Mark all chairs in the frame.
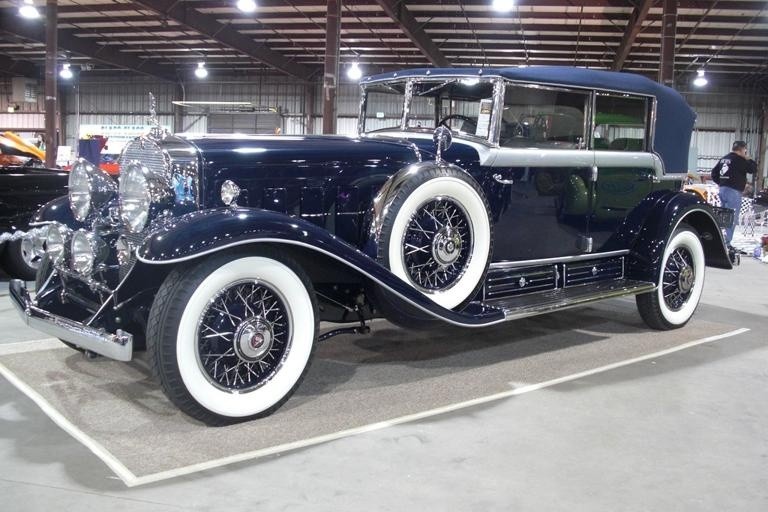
[706,187,768,237]
[462,106,587,146]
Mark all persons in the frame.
[37,133,47,152]
[711,141,757,247]
